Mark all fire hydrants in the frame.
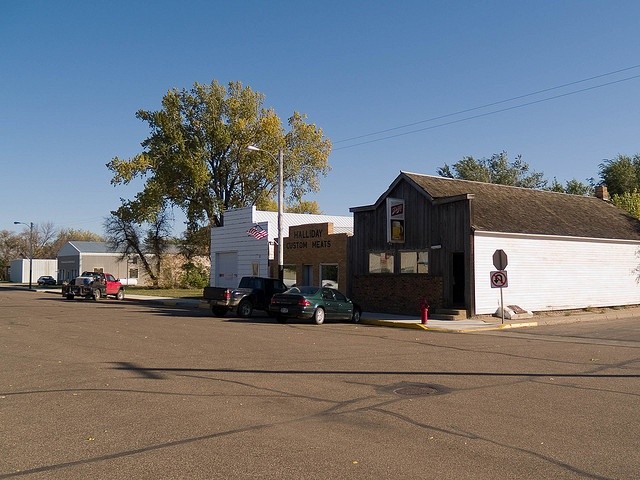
[419,298,430,323]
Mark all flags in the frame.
[247,225,268,240]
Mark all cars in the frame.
[270,286,362,325]
[38,276,56,285]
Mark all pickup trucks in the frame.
[62,271,125,300]
[204,277,287,317]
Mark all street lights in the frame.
[247,145,286,291]
[14,222,32,289]
